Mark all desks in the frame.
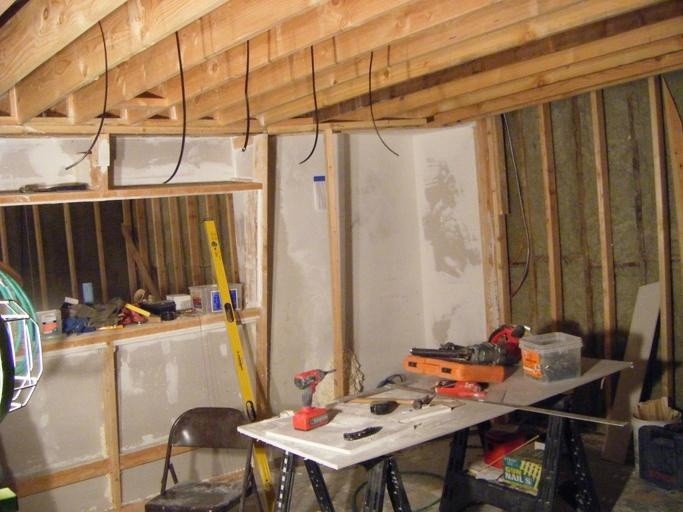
[236,340,632,509]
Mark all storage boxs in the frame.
[518,329,584,381]
[402,352,507,385]
[166,293,193,312]
[188,280,242,313]
[500,434,556,490]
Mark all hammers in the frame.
[345,397,423,410]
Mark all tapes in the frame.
[161,311,175,320]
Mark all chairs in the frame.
[141,404,255,510]
